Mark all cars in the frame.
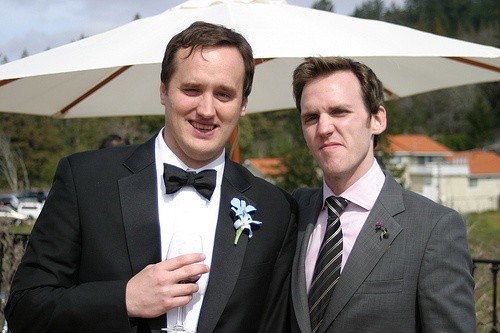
[0,190,49,227]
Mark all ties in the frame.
[308,196,350,333]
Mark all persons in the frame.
[283,56,477,333]
[4,20,299,333]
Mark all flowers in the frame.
[230,197,262,245]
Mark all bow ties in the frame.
[163,163,217,201]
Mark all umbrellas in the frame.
[0,0,500,164]
